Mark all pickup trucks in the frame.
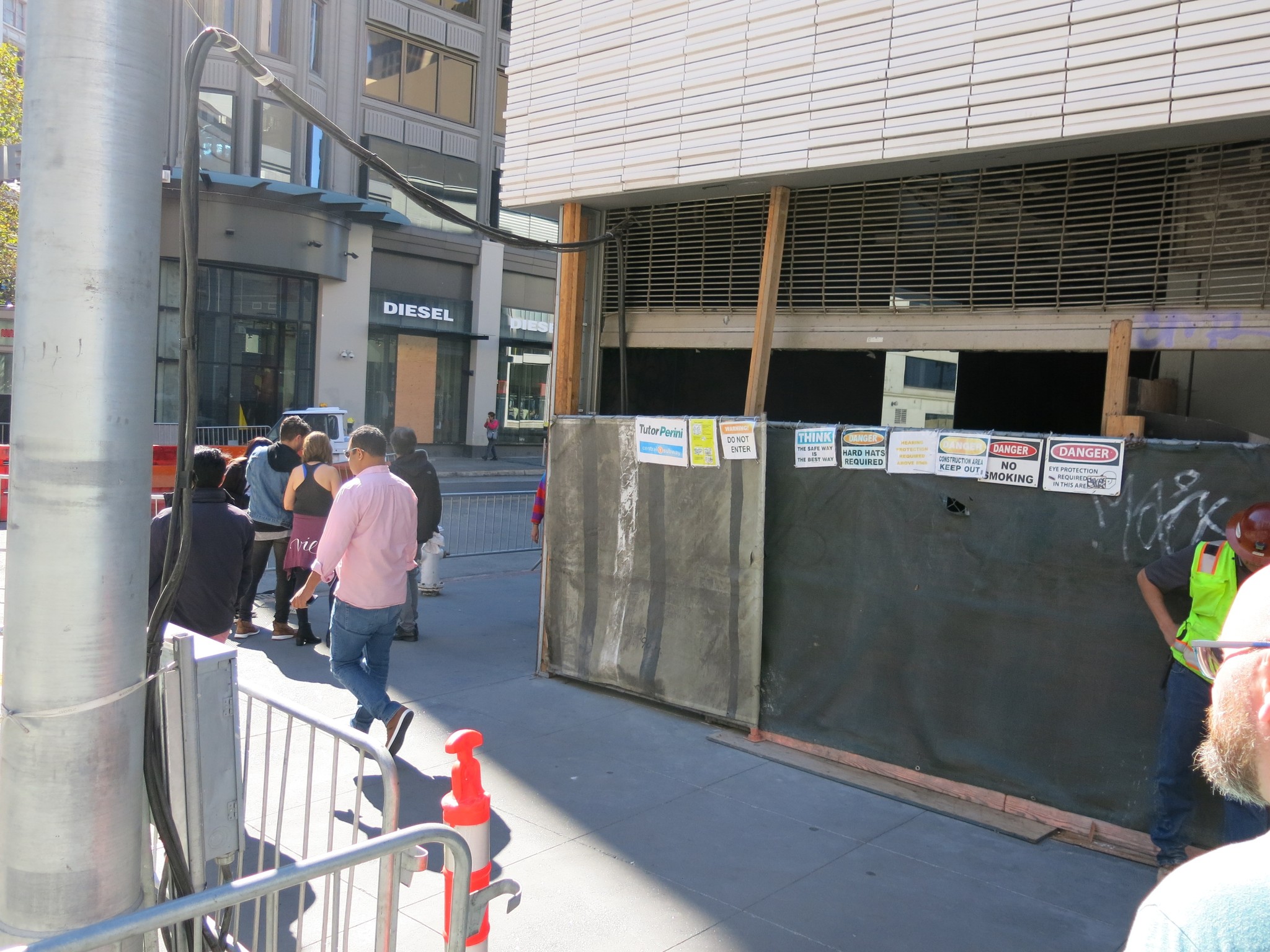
[262,404,353,467]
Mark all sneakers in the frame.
[482,456,487,460]
[272,620,299,640]
[1157,863,1183,883]
[288,605,297,615]
[385,705,414,756]
[490,457,498,461]
[234,618,260,637]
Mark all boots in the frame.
[296,621,322,646]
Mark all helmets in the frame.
[1225,502,1270,568]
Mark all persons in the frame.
[1133,503,1270,878]
[283,432,344,648]
[481,412,500,462]
[530,462,546,545]
[1123,562,1268,952]
[217,437,275,509]
[386,427,442,641]
[289,423,418,760]
[230,414,311,640]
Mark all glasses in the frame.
[1191,641,1270,680]
[343,448,365,458]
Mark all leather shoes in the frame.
[392,624,418,642]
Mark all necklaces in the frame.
[145,445,255,644]
